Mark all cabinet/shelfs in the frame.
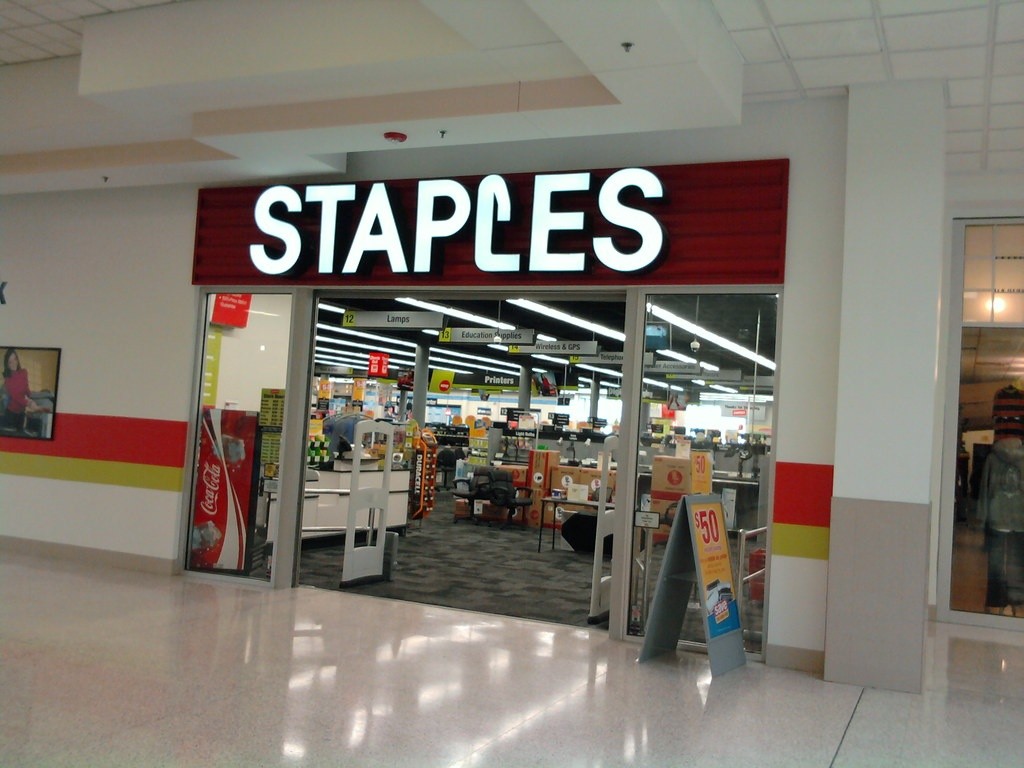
[435,434,471,457]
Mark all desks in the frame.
[538,495,615,554]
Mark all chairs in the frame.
[434,447,458,492]
[451,466,500,526]
[488,470,534,531]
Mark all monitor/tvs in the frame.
[645,321,672,351]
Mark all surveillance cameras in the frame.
[691,342,700,352]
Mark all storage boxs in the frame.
[749,548,766,601]
[455,450,691,543]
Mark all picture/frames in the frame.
[0,345,61,441]
